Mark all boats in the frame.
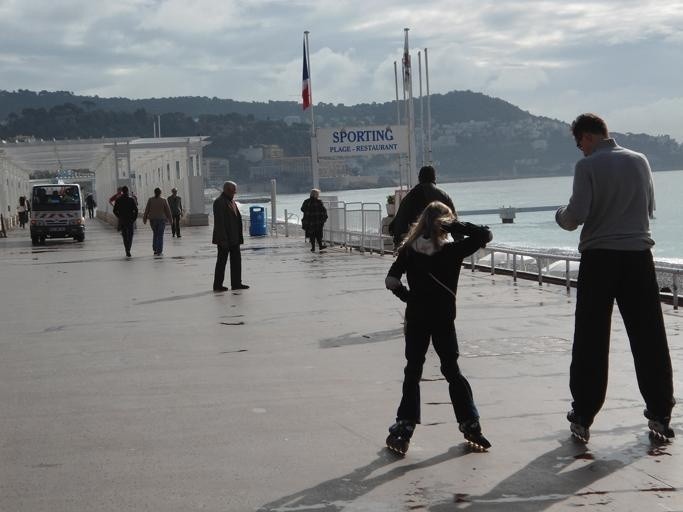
[235,197,271,203]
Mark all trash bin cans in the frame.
[249,205,267,238]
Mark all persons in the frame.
[387,166,458,253]
[210,180,250,292]
[15,188,96,228]
[300,188,328,251]
[381,199,494,443]
[107,184,183,259]
[554,112,677,428]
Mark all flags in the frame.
[300,37,311,110]
[401,29,412,94]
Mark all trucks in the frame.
[18,180,93,243]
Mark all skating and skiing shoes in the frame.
[644,410,675,438]
[567,410,594,441]
[386,433,409,457]
[464,432,490,452]
